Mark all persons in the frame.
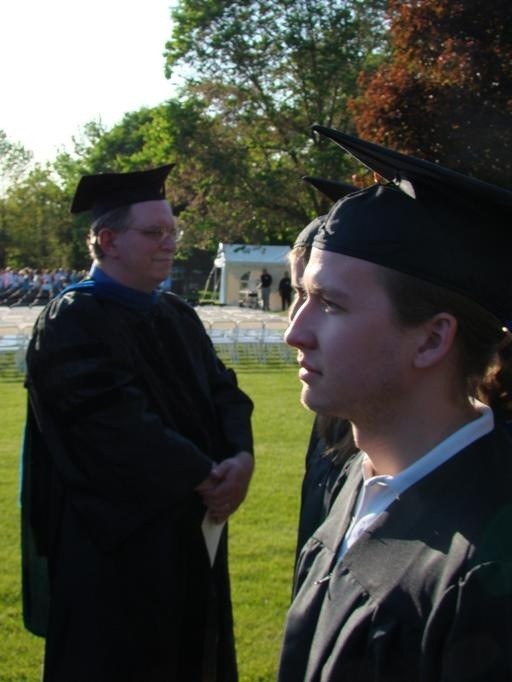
[0,267,199,307]
[275,125,510,679]
[260,267,272,310]
[279,271,294,310]
[288,176,366,599]
[23,163,254,680]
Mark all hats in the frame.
[71,164,174,213]
[292,176,362,248]
[312,125,511,319]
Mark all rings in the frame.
[226,504,231,511]
